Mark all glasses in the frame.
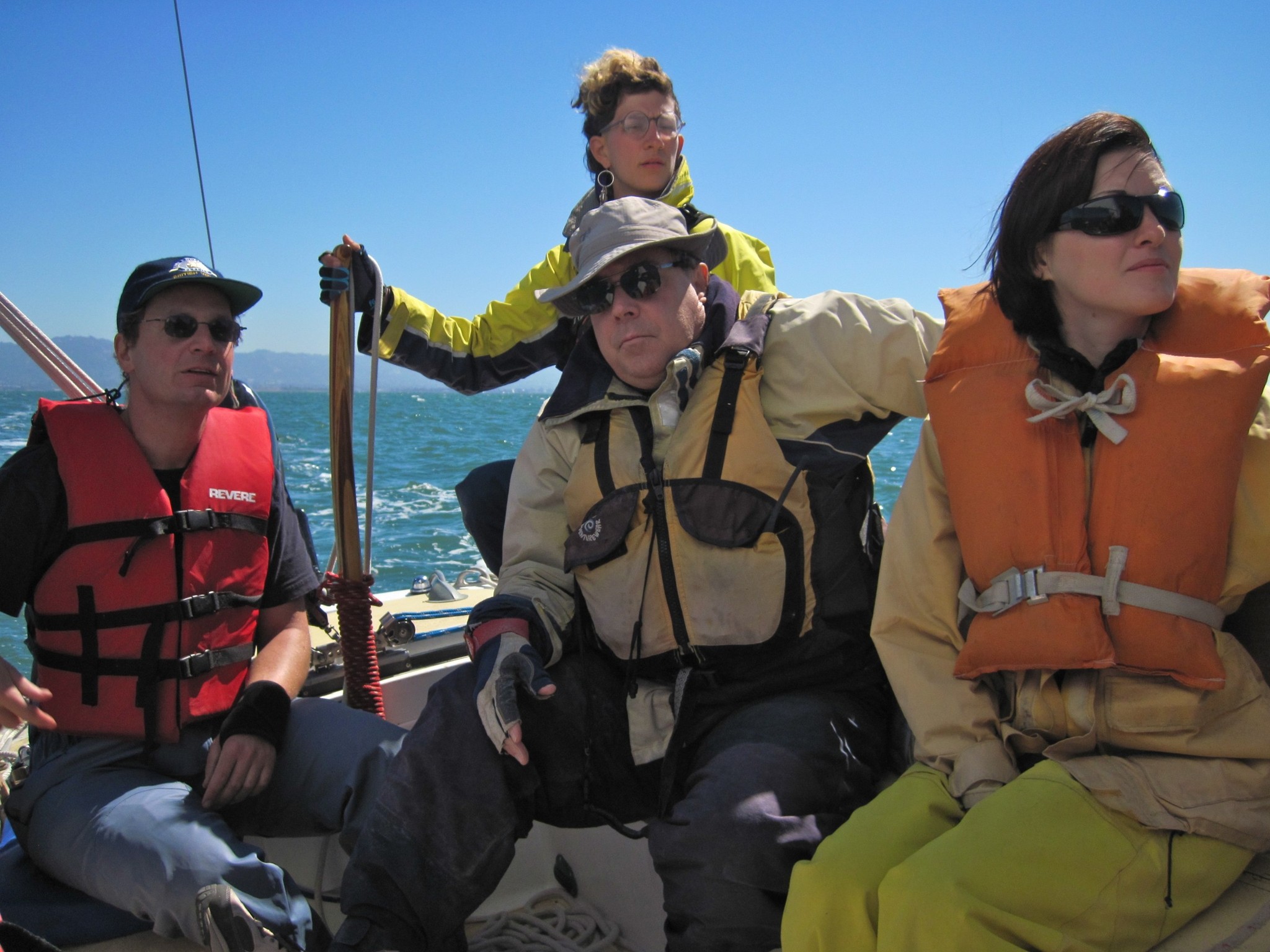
[1057,191,1184,236]
[139,313,247,344]
[598,110,687,140]
[575,260,693,315]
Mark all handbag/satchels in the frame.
[488,643,655,829]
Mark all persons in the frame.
[338,194,945,952]
[780,109,1270,951]
[318,45,775,579]
[0,256,470,951]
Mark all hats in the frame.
[115,255,261,331]
[532,195,727,304]
